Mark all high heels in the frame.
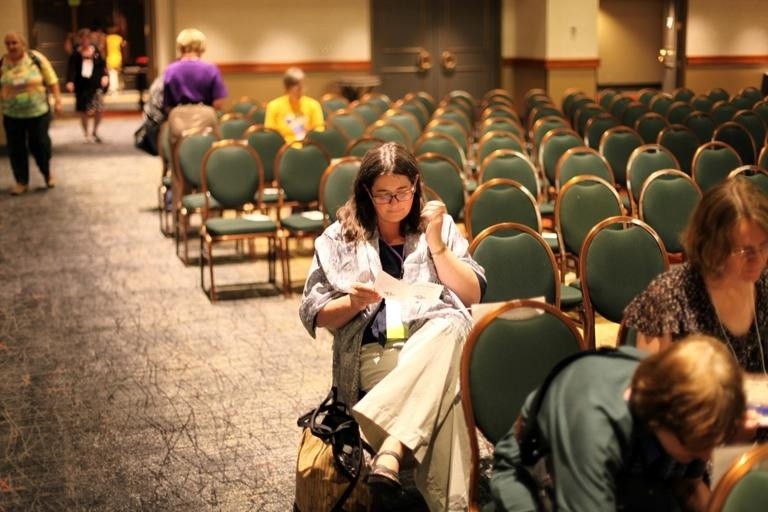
[360,449,405,495]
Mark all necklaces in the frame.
[707,285,768,380]
[379,228,412,274]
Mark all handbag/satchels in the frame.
[517,415,557,511]
[292,384,370,512]
[133,102,164,156]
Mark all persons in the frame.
[142,73,173,211]
[64,10,128,142]
[484,335,746,512]
[0,33,63,195]
[266,66,324,150]
[621,176,768,445]
[299,142,487,511]
[162,28,228,111]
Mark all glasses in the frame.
[724,240,767,259]
[359,174,417,208]
